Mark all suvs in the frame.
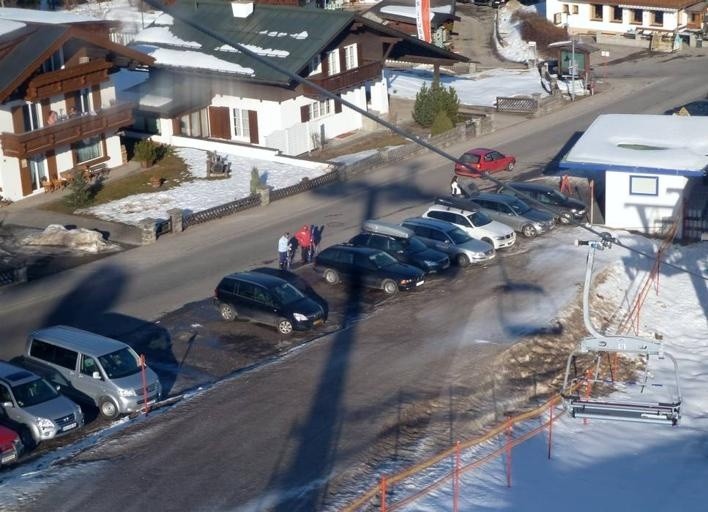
[0,360,85,451]
[213,270,325,334]
[301,182,589,293]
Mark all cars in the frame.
[0,424,23,471]
[454,148,516,178]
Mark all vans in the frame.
[23,325,162,421]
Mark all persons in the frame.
[294,224,315,264]
[277,231,292,271]
[449,176,463,199]
